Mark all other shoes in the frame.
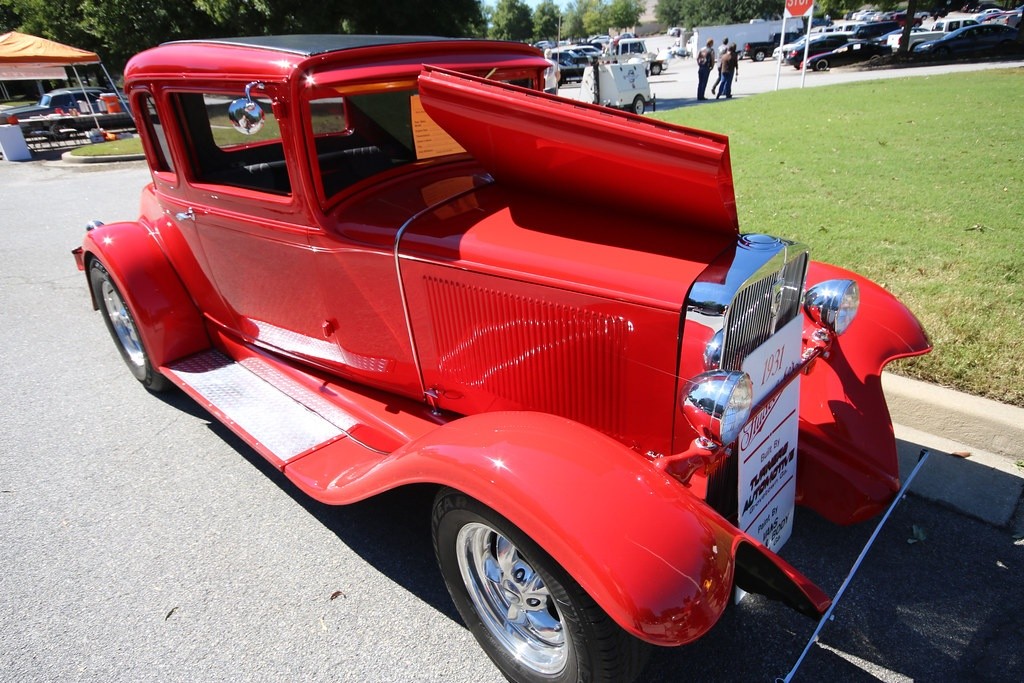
[726,96,732,98]
[712,89,715,94]
[716,97,719,99]
[703,98,708,100]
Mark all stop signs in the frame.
[784,0,814,17]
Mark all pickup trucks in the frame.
[743,31,804,62]
[886,15,985,59]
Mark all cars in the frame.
[508,27,692,86]
[0,86,160,142]
[69,34,942,683]
[912,23,1024,62]
[772,7,1024,52]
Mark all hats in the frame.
[544,49,552,59]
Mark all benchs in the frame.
[237,146,385,198]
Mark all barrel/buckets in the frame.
[99,93,122,112]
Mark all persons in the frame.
[695,37,738,101]
[542,49,562,96]
[974,28,983,37]
[239,115,253,133]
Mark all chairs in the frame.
[55,119,78,148]
[28,121,54,152]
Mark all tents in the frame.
[0,31,136,133]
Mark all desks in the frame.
[18,112,125,151]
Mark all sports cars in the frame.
[806,40,893,71]
